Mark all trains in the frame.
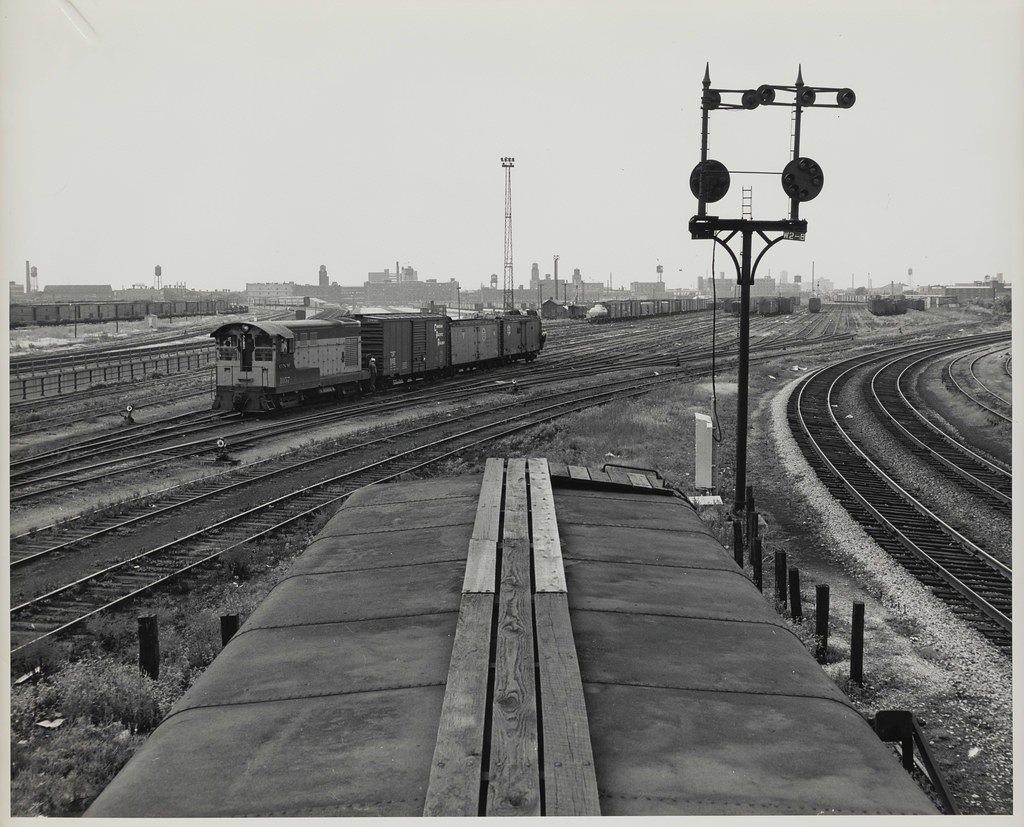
[208,298,548,422]
